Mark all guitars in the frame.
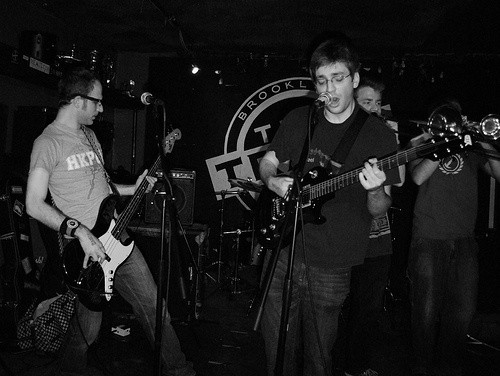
[254,123,481,250]
[59,124,182,310]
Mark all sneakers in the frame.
[344,368,380,376]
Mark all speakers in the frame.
[143,169,197,226]
[0,195,22,306]
[125,221,210,322]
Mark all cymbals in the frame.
[226,177,266,193]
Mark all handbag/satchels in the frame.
[16,289,80,352]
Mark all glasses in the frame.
[71,93,102,106]
[312,74,351,87]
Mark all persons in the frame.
[354,80,406,376]
[258,39,402,376]
[25,71,198,376]
[406,101,500,376]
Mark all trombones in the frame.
[376,101,500,157]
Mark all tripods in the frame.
[205,187,268,295]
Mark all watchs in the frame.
[66,219,78,235]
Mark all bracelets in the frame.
[60,216,81,237]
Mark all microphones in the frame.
[140,92,164,106]
[315,92,333,106]
